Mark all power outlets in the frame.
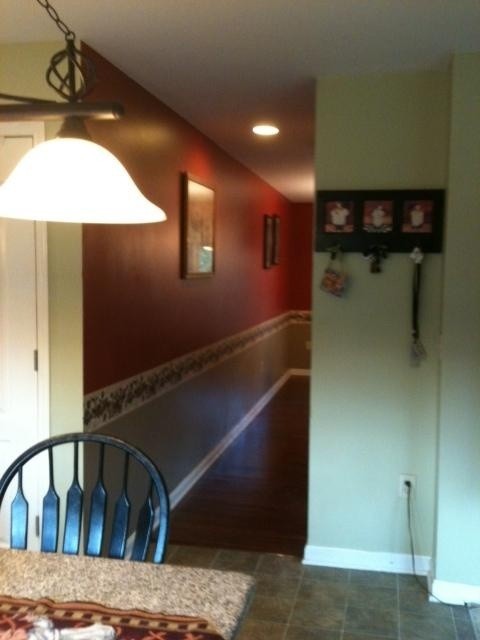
[398,474,415,495]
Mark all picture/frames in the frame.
[263,214,276,268]
[179,171,216,280]
[273,220,281,264]
[314,187,446,255]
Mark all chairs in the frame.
[0,434,167,566]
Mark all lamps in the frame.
[0,137,168,227]
